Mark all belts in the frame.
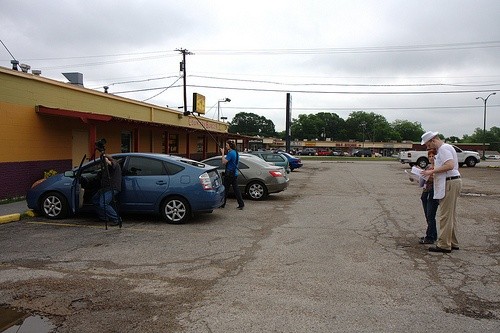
[446,176,460,180]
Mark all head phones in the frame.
[230,140,234,147]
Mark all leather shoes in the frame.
[429,246,460,253]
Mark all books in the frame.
[410,165,430,182]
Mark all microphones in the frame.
[184,111,193,116]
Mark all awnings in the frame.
[35,104,264,141]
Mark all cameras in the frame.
[96,138,107,146]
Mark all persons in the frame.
[92,155,122,227]
[217,140,246,210]
[418,132,462,252]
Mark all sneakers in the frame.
[93,216,109,221]
[109,219,122,226]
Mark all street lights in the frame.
[475,92,497,160]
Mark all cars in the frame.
[250,151,303,172]
[274,147,399,157]
[487,155,500,159]
[199,153,290,199]
[24,152,224,222]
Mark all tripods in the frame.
[96,146,123,230]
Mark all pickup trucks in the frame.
[400,145,481,167]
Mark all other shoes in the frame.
[219,204,225,208]
[237,204,244,208]
[418,237,434,244]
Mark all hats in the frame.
[420,132,438,145]
